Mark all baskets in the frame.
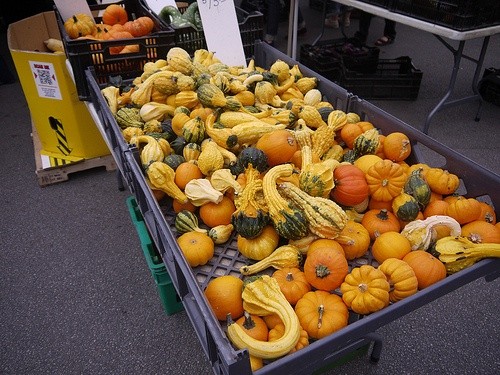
[300,39,381,80]
[144,0,265,67]
[74,56,500,372]
[325,56,423,102]
[54,0,177,102]
[389,0,500,32]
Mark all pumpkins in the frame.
[101,47,500,371]
[44,3,198,71]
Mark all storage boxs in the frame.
[6,0,500,375]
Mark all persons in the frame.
[262,0,308,49]
[354,0,395,45]
[324,0,351,29]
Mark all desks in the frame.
[329,0,500,136]
